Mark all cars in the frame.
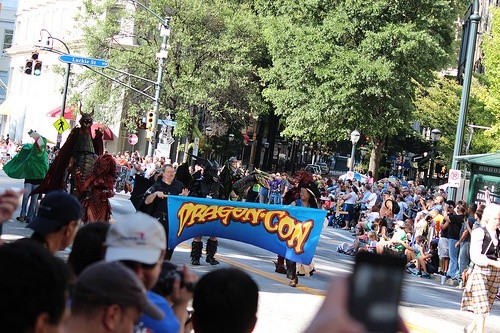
[303,161,330,176]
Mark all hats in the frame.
[396,220,406,228]
[25,191,82,231]
[104,213,168,264]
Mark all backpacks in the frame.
[385,200,399,214]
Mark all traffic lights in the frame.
[24,59,33,75]
[33,60,42,76]
[146,110,155,129]
[31,51,39,60]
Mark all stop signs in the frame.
[128,133,138,145]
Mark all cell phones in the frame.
[348,251,406,333]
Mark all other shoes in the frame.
[422,273,430,278]
[16,216,24,222]
[27,218,32,223]
[342,227,350,229]
[412,271,422,277]
[276,266,287,274]
[347,251,356,256]
[290,280,296,286]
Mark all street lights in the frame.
[426,128,442,190]
[349,128,360,172]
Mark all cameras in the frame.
[150,260,183,297]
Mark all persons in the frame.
[0,110,500,333]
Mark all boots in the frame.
[190,241,203,266]
[205,238,219,265]
